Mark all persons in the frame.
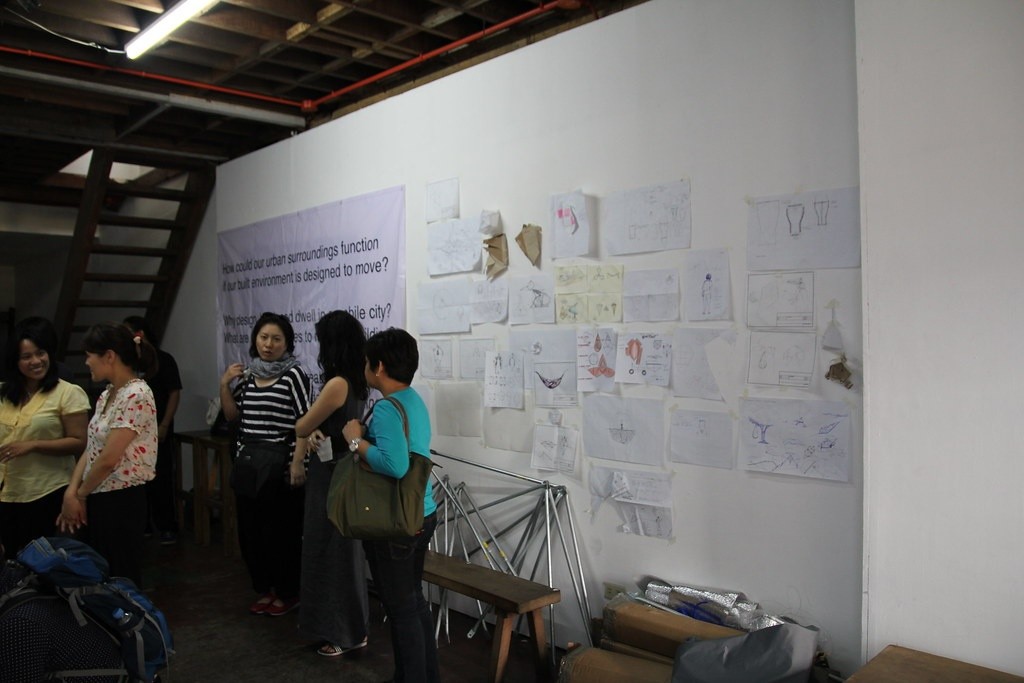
[343,326,441,681]
[56,315,181,584]
[220,313,321,616]
[295,308,372,655]
[0,316,92,569]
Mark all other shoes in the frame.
[249,591,278,613]
[265,597,302,617]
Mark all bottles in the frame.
[112,608,132,625]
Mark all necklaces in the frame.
[21,383,40,397]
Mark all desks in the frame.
[173,430,241,558]
[843,645,1024,683]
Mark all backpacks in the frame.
[16,535,175,683]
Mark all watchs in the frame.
[348,436,363,453]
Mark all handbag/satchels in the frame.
[325,398,444,540]
[228,444,293,506]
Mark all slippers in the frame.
[317,636,368,656]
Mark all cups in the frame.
[316,437,333,463]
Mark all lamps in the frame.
[124,0,219,61]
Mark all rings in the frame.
[7,452,10,455]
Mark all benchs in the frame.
[421,550,561,683]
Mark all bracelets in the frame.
[308,437,312,442]
[77,497,87,501]
